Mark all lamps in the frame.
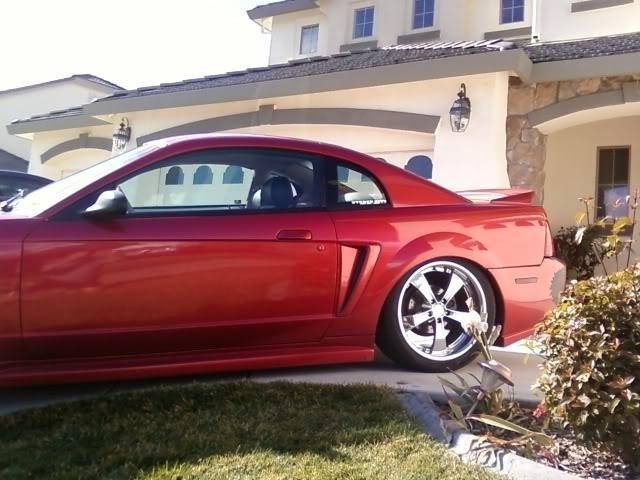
[112,116,131,154]
[449,83,471,132]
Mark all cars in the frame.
[0,170,55,203]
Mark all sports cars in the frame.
[0,133,568,385]
